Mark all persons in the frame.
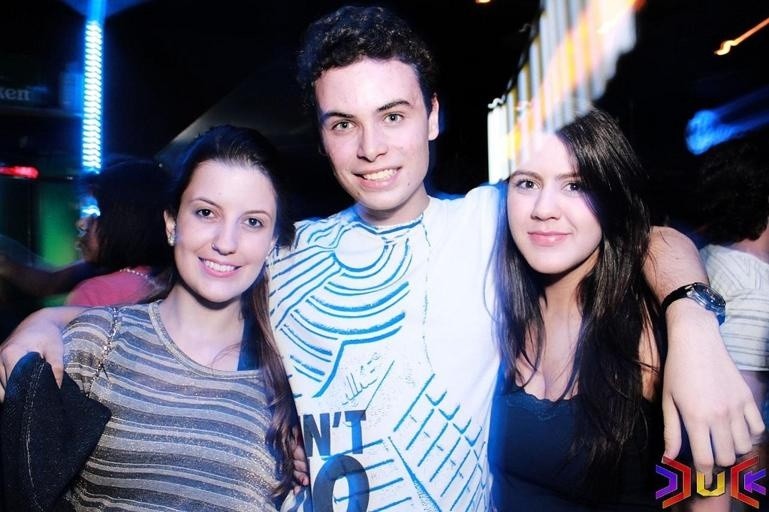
[0,7,769,512]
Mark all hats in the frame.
[3,352,112,511]
